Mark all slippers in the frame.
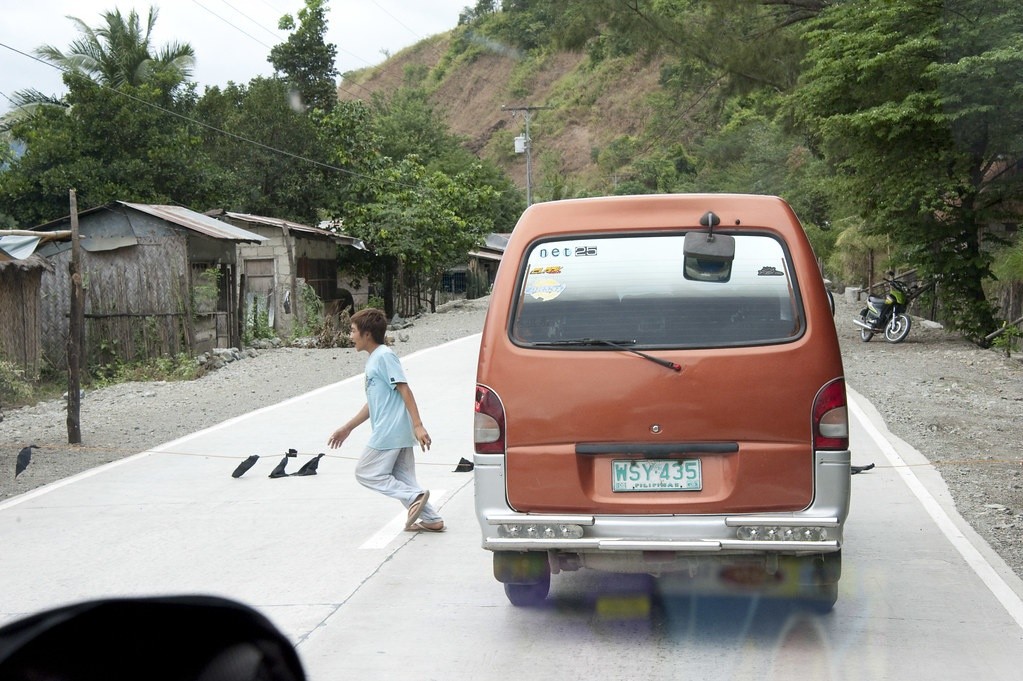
[409,523,448,532]
[408,490,430,529]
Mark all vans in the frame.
[472,192,852,616]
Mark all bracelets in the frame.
[413,423,422,429]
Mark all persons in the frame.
[327,308,450,534]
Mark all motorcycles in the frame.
[852,270,919,344]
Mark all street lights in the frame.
[513,135,533,209]
